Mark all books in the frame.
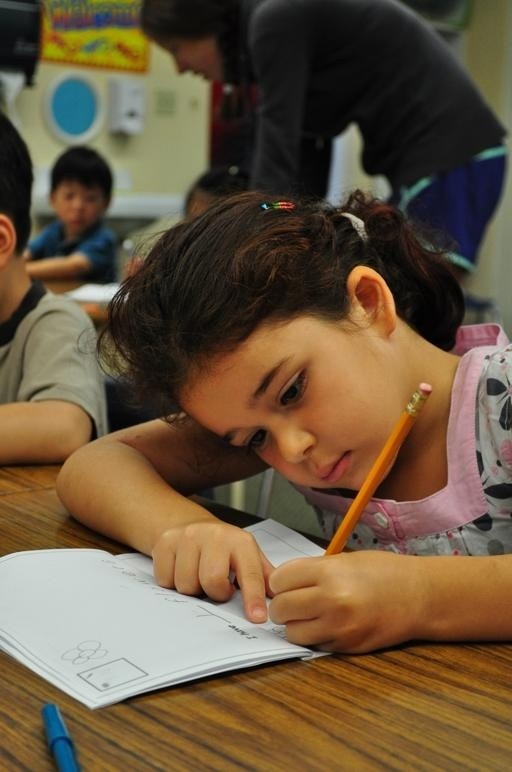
[0,517,332,713]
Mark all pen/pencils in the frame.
[41,703,81,772]
[325,382,432,555]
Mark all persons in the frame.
[54,188,512,656]
[0,112,109,466]
[22,146,120,284]
[124,167,247,277]
[138,0,510,292]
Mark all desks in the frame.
[44,282,109,327]
[1,463,512,772]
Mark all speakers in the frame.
[0,1,41,68]
